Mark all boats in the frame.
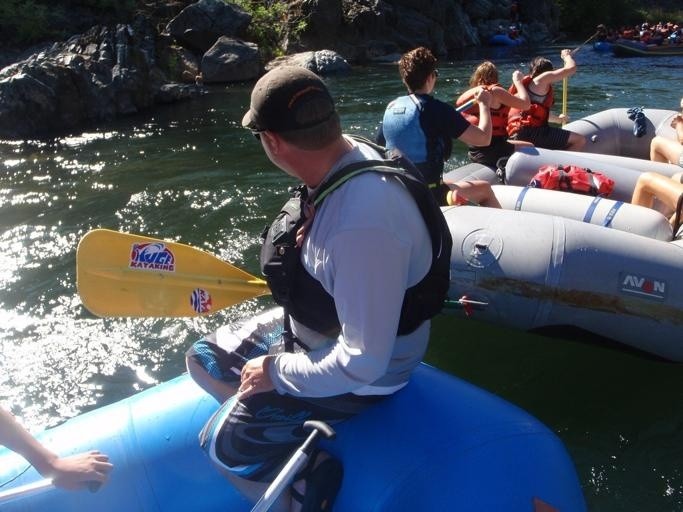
[0,356,587,512]
[487,31,528,48]
[437,104,683,364]
[592,36,682,57]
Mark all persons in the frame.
[374,47,501,213]
[648,114,682,167]
[456,55,530,166]
[185,63,456,511]
[496,20,525,41]
[589,16,683,51]
[0,402,112,495]
[628,168,682,231]
[499,46,587,155]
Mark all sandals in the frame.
[290,448,343,512]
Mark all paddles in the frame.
[75,229,490,318]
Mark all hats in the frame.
[242,65,335,132]
